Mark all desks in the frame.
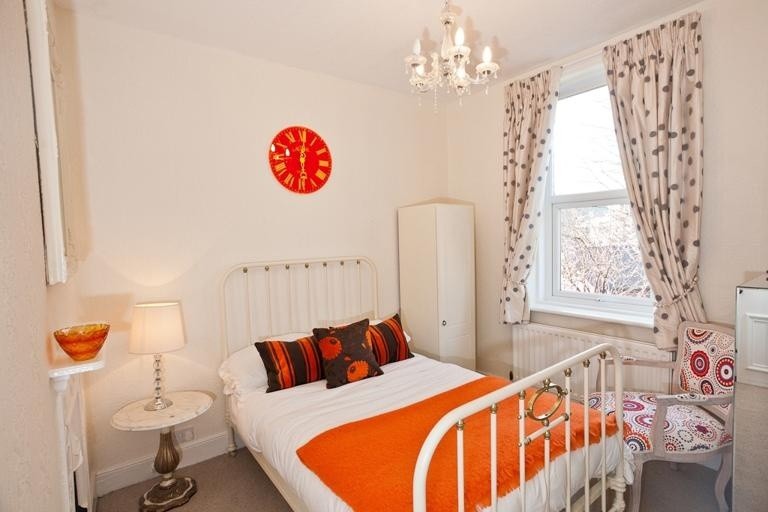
[108,391,214,512]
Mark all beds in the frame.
[219,256,626,512]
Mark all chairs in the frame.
[579,321,736,512]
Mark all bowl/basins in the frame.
[53,323,110,363]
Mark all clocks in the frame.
[269,126,332,194]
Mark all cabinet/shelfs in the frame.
[397,203,476,372]
[731,271,768,512]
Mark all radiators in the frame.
[512,322,676,401]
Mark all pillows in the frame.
[255,336,326,393]
[369,318,411,343]
[312,318,384,389]
[368,313,415,367]
[218,333,310,403]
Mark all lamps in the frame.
[128,303,185,411]
[400,0,500,115]
